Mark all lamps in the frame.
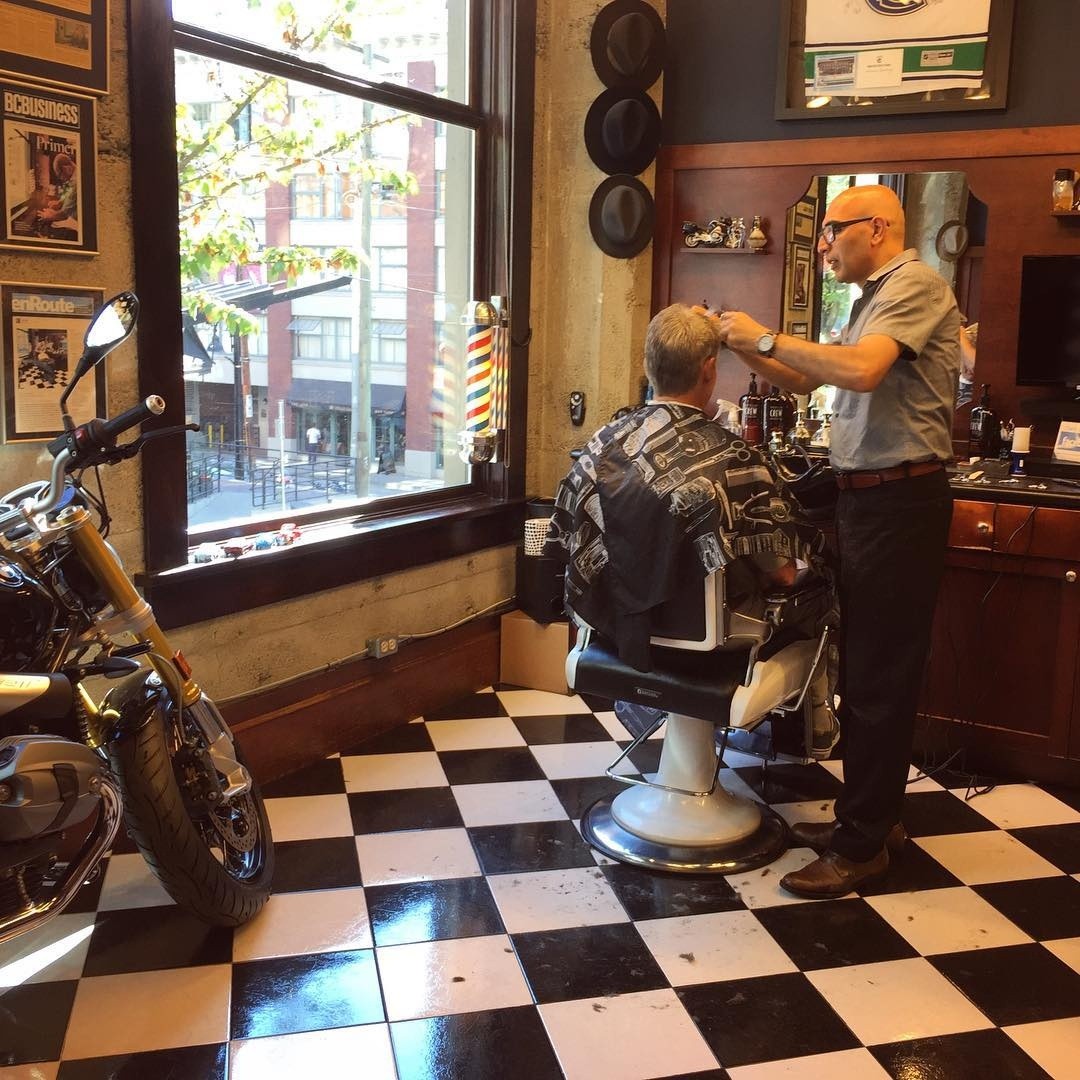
[428,293,467,458]
[464,295,508,467]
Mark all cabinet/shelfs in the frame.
[909,499,1080,786]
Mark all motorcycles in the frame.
[0,294,273,991]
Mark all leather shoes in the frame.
[789,822,905,858]
[778,840,889,898]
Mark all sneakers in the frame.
[808,709,841,761]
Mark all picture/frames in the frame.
[0,76,101,256]
[775,0,1014,121]
[0,280,109,445]
[0,0,111,97]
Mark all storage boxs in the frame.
[500,609,579,697]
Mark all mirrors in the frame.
[778,170,989,421]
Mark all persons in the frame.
[685,182,970,902]
[305,420,323,466]
[621,299,842,761]
[36,152,80,234]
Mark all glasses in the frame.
[817,217,891,245]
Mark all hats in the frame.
[583,87,663,177]
[589,0,667,98]
[588,173,656,259]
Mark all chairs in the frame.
[563,474,837,876]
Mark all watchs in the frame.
[754,328,783,362]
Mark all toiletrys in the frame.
[737,371,794,458]
[967,384,1000,463]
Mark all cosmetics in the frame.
[736,370,763,444]
[761,384,789,443]
[965,381,999,457]
